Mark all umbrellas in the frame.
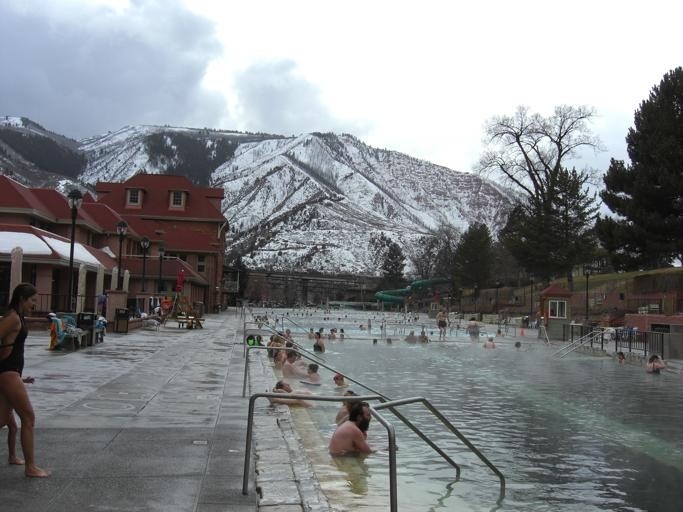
[175,268,184,293]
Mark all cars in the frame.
[585,326,641,341]
[638,303,660,314]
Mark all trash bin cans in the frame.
[194,301,203,317]
[77,312,95,346]
[235,302,240,307]
[113,308,130,333]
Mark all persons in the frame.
[617,351,625,364]
[264,348,398,459]
[250,300,541,348]
[0,374,35,465]
[0,282,50,476]
[646,354,665,374]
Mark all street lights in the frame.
[474,282,476,315]
[458,288,463,314]
[117,221,128,291]
[66,190,84,312]
[158,246,165,291]
[528,273,535,317]
[494,279,499,307]
[140,235,150,292]
[582,264,591,321]
